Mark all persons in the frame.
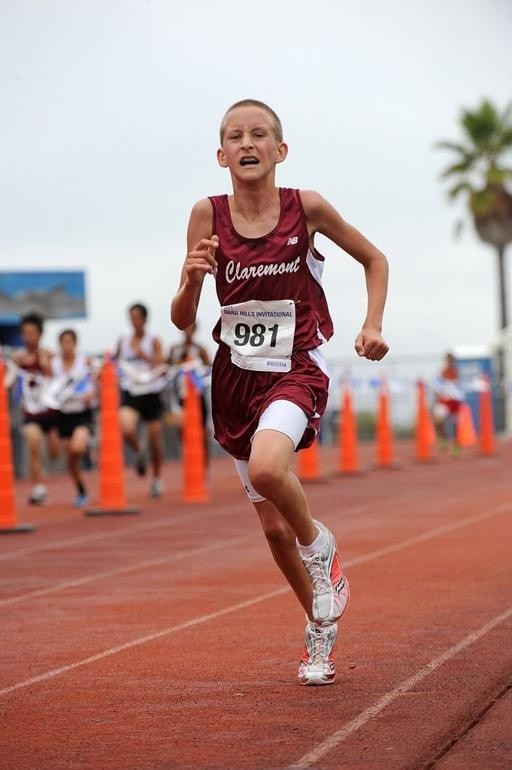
[167,321,211,483]
[2,313,63,508]
[110,304,166,498]
[433,352,465,458]
[40,327,97,508]
[171,98,389,684]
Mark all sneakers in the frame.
[22,445,166,510]
[293,517,352,623]
[293,616,343,688]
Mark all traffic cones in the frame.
[335,386,366,479]
[0,354,35,533]
[452,399,477,449]
[298,433,332,484]
[478,381,499,457]
[409,377,442,466]
[79,353,141,516]
[178,372,212,503]
[372,391,400,471]
